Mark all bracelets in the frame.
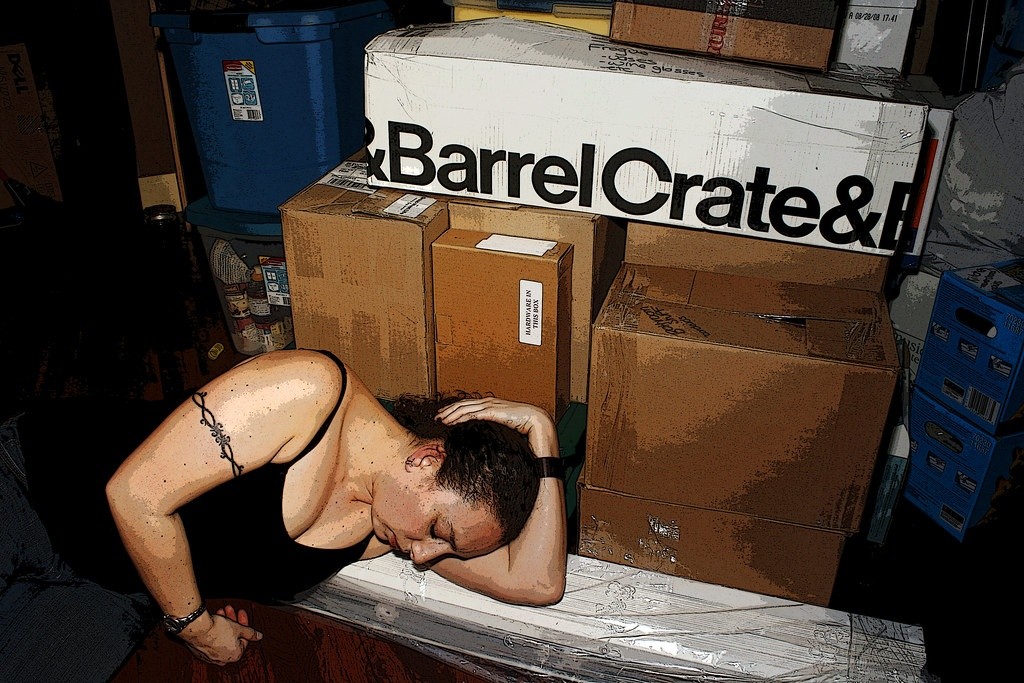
[535,457,562,482]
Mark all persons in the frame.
[0,347,568,683]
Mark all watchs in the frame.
[162,601,206,634]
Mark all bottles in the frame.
[248,273,276,322]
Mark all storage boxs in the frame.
[610,1,840,72]
[625,222,889,293]
[0,42,72,211]
[185,198,296,354]
[150,1,399,214]
[365,18,928,256]
[903,386,1024,541]
[835,0,917,74]
[868,368,911,545]
[444,0,612,39]
[448,198,626,405]
[279,145,448,403]
[585,263,900,534]
[576,468,846,607]
[914,261,1024,436]
[431,229,574,427]
